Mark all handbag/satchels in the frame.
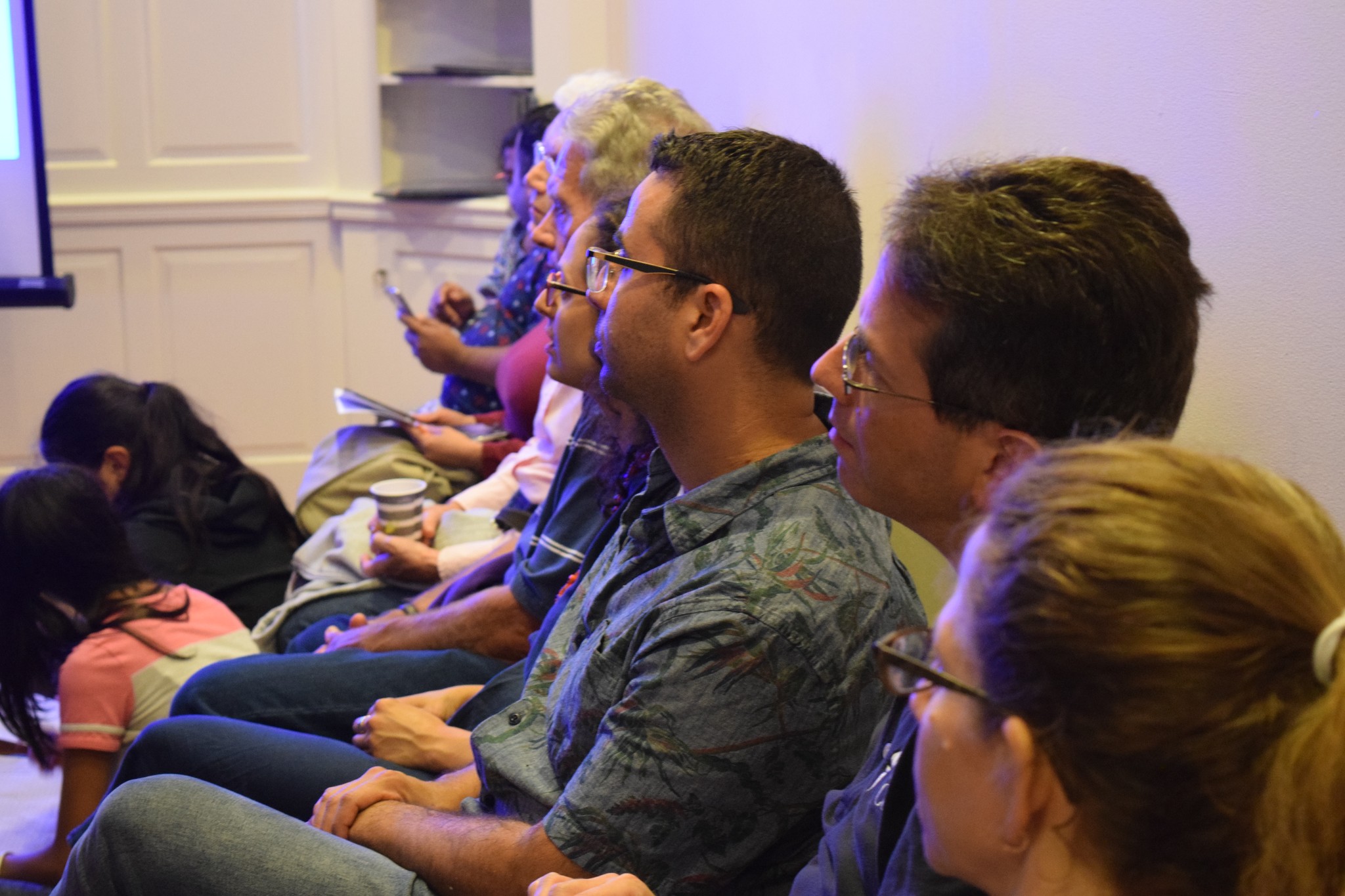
[294,423,478,538]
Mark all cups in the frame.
[369,477,427,543]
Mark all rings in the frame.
[358,716,365,733]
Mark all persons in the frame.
[0,462,264,896]
[48,129,932,896]
[39,373,311,634]
[65,71,717,847]
[522,153,1215,896]
[907,434,1345,896]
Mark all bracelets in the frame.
[396,601,418,615]
[0,848,15,869]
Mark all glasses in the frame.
[842,333,983,415]
[546,272,586,305]
[533,142,555,175]
[872,627,1004,710]
[585,247,750,316]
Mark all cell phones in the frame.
[387,287,414,317]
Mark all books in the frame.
[333,386,435,428]
